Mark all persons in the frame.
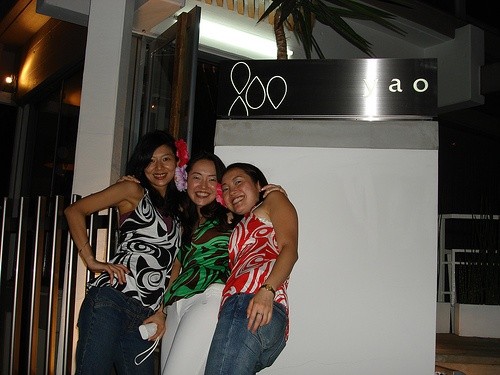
[117,152,287,375]
[65,129,184,375]
[204,163,298,375]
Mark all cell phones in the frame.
[139,323,158,339]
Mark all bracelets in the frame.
[79,242,88,253]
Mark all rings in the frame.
[257,312,264,316]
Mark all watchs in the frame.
[261,284,275,295]
